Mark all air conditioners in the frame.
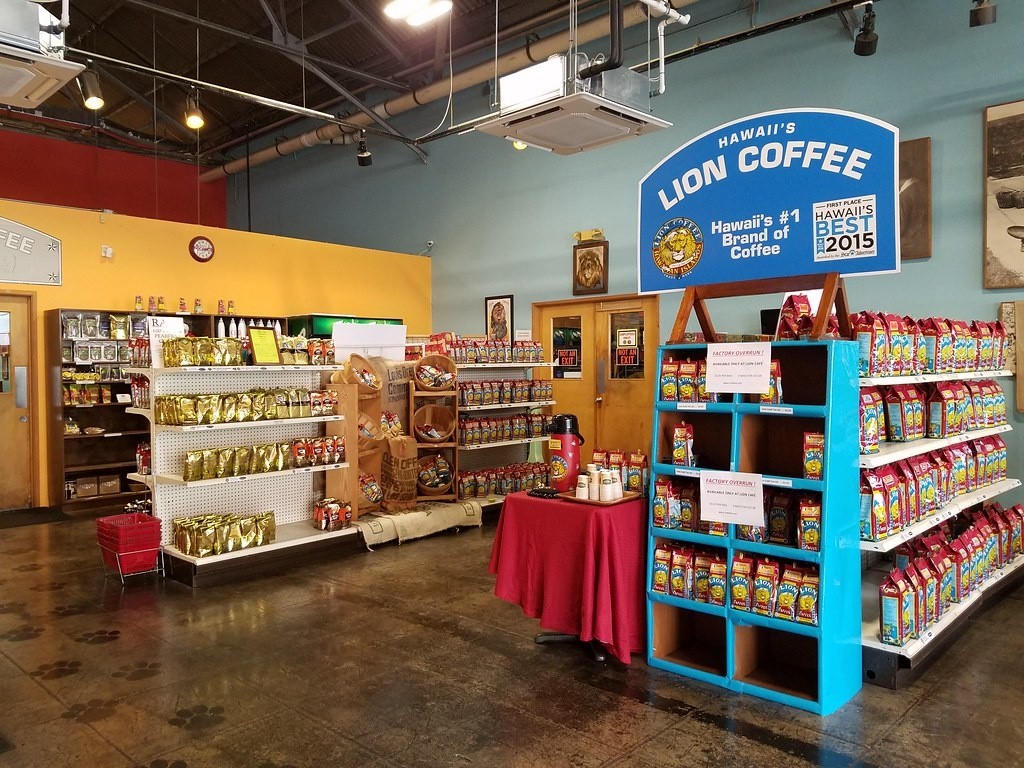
[0,0,86,108]
[475,55,673,156]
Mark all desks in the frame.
[504,487,650,660]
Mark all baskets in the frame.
[96,512,164,574]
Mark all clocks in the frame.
[189,235,215,262]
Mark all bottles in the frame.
[229,318,237,337]
[218,318,225,338]
[238,318,281,338]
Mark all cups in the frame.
[576,464,624,503]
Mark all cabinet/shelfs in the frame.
[43,308,288,511]
[648,340,1024,715]
[122,342,557,586]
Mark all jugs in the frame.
[546,414,585,492]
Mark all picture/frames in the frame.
[573,240,609,294]
[983,98,1024,289]
[485,294,514,347]
[899,136,931,259]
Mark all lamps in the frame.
[75,60,105,109]
[357,129,372,166]
[853,1,879,56]
[970,0,996,27]
[184,84,205,128]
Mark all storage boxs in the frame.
[96,514,162,573]
